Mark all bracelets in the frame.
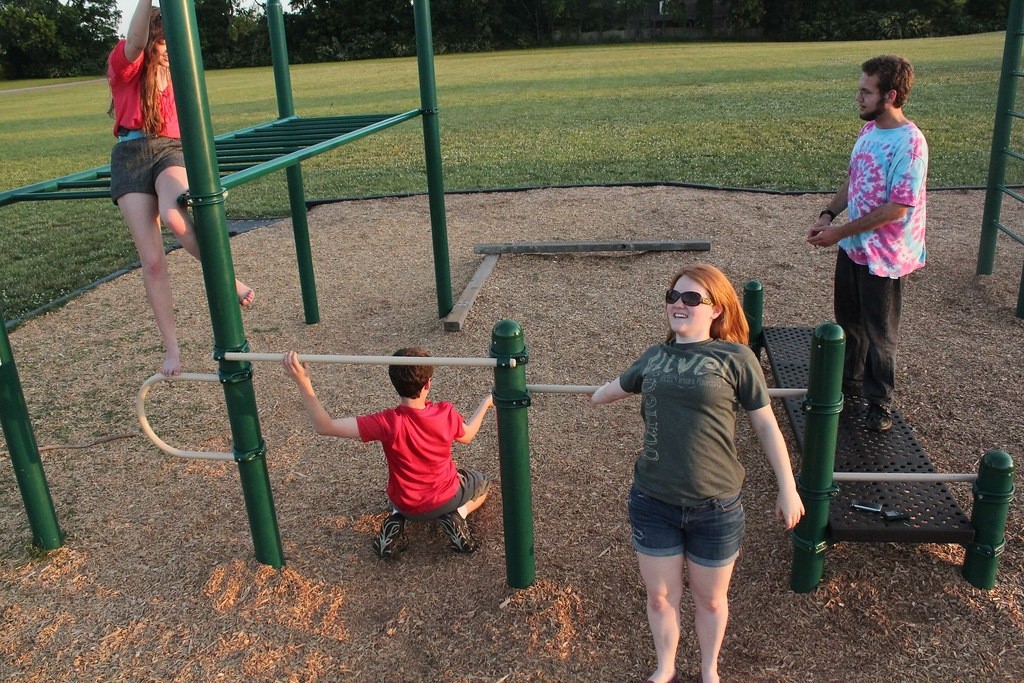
[818,210,835,222]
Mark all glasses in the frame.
[666,290,714,306]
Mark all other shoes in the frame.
[698,671,722,683]
[644,668,677,683]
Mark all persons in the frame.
[586,262,808,683]
[101,0,258,379]
[798,49,931,434]
[279,344,498,559]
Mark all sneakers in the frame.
[437,511,476,553]
[373,513,405,558]
[866,400,892,430]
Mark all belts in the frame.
[119,129,145,142]
